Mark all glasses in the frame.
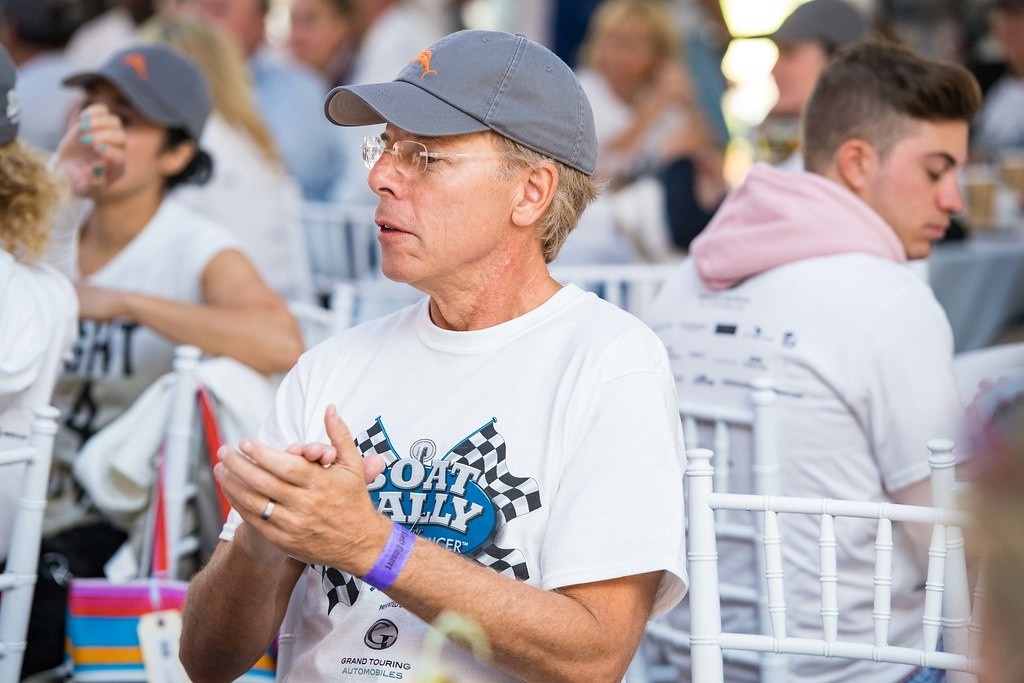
[361,136,502,176]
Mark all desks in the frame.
[927,236,1024,352]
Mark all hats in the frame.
[766,0,863,51]
[0,45,21,146]
[63,45,208,141]
[323,29,598,177]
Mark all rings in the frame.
[263,500,275,518]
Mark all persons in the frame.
[0,0,1024,683]
[178,29,689,683]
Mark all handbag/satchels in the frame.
[66,387,278,683]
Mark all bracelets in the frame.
[364,521,415,589]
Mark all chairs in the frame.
[0,180,983,683]
